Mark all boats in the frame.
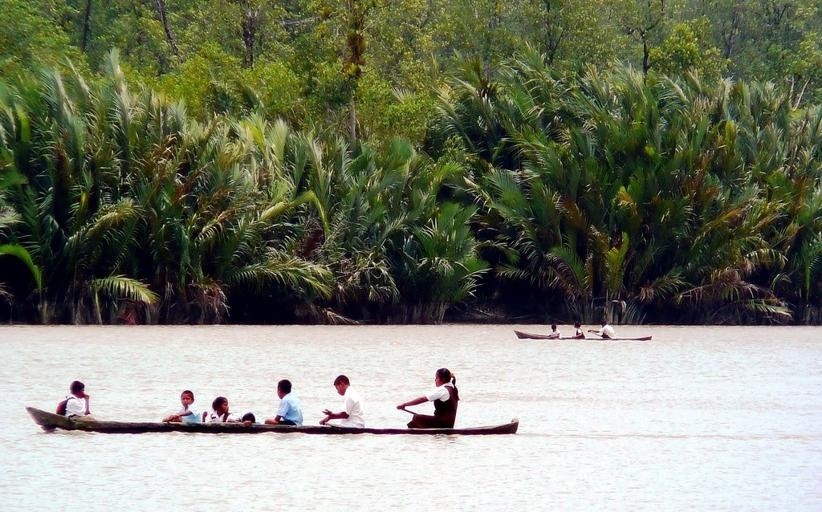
[27,407,519,434]
[515,331,652,340]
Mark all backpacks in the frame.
[56,396,75,416]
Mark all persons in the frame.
[57,381,96,421]
[397,367,460,428]
[573,321,585,339]
[162,390,202,422]
[318,375,365,429]
[202,396,237,422]
[264,380,303,425]
[548,324,561,339]
[242,412,261,425]
[590,318,616,338]
